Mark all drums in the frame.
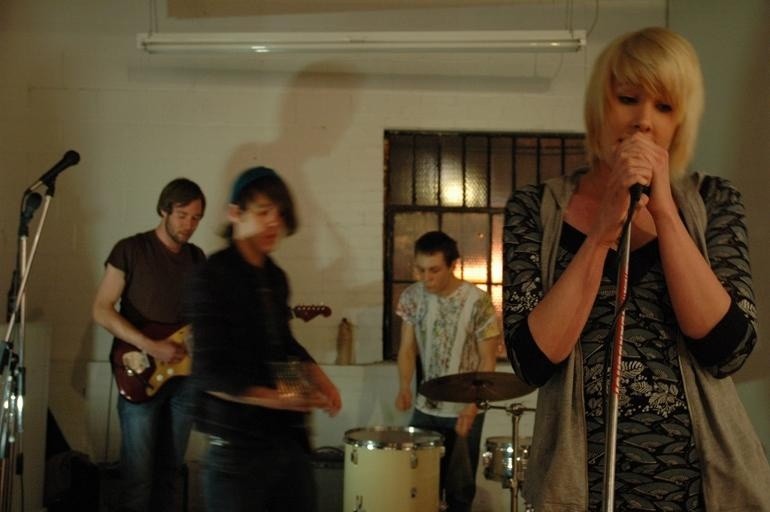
[340,424,447,511]
[481,434,536,490]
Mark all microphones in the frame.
[17,192,42,237]
[23,149,81,194]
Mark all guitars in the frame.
[106,300,334,407]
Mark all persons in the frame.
[395,228,501,510]
[502,24,760,509]
[91,177,206,511]
[197,166,342,510]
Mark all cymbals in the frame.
[416,369,538,405]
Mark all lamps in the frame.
[139,30,589,56]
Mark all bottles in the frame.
[336,317,352,366]
